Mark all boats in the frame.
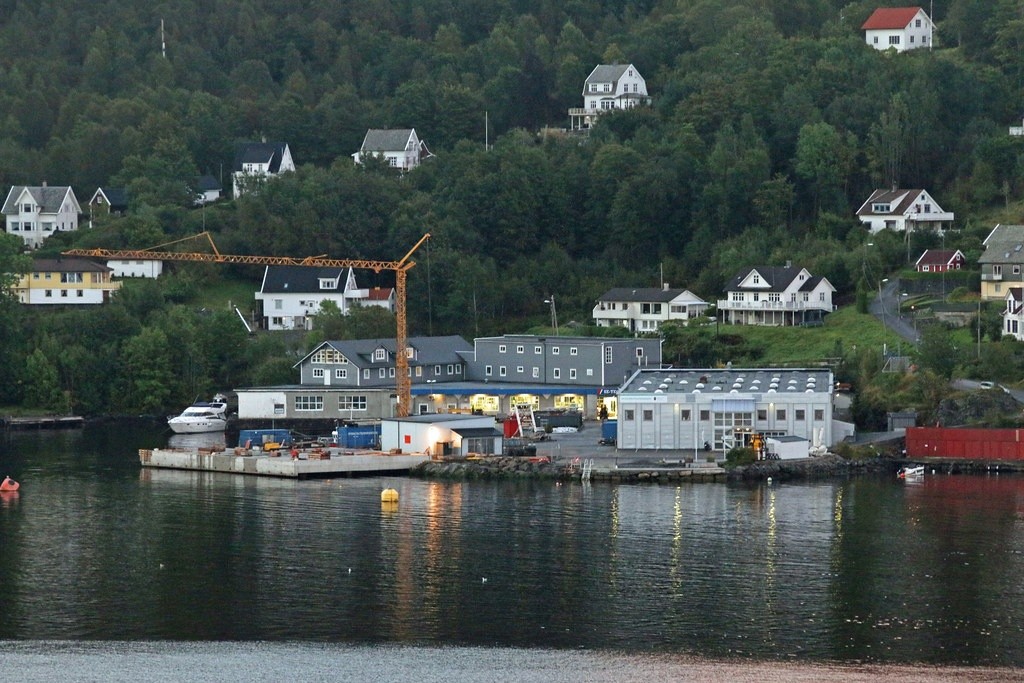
[167,394,227,433]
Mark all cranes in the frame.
[61,233,429,418]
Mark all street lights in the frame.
[879,279,888,325]
[544,300,555,334]
[897,294,907,326]
[862,242,873,263]
